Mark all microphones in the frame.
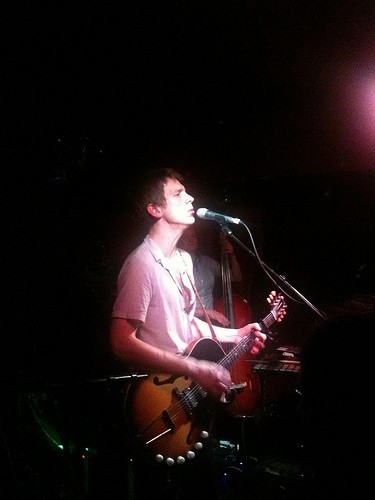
[196,208,242,225]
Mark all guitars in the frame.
[121,289,290,465]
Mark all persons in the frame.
[108,166,266,398]
[176,225,243,328]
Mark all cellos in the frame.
[197,195,265,421]
